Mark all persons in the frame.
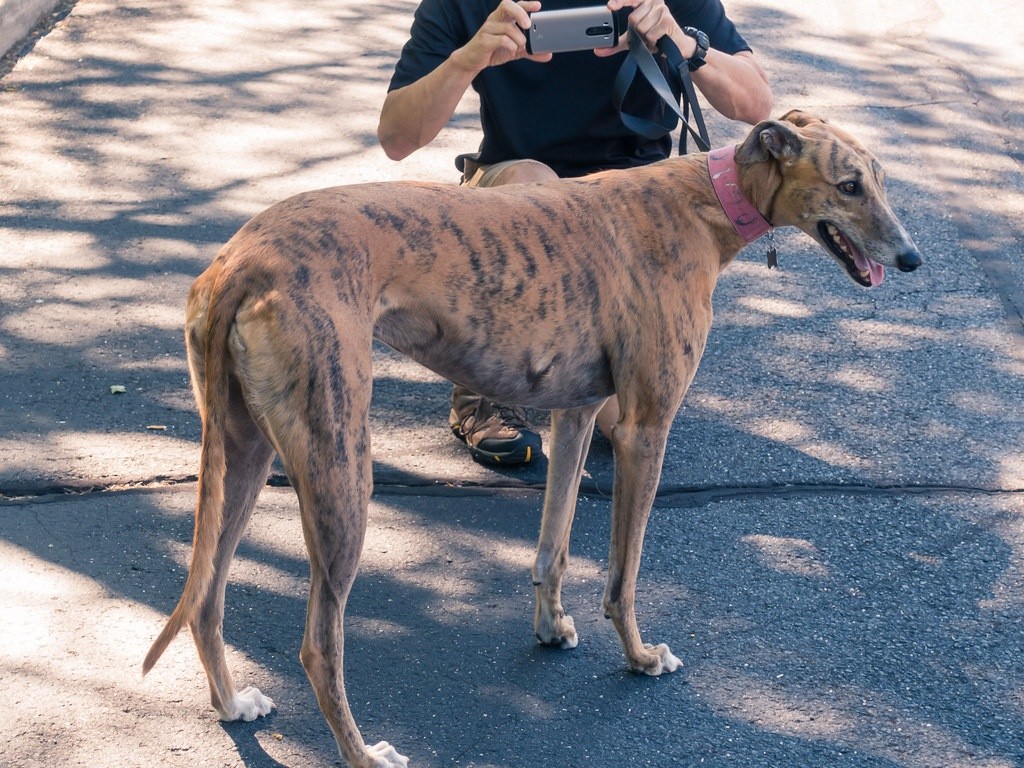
[378,0,771,467]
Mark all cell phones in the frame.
[526,5,620,55]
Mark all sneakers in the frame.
[448,384,542,465]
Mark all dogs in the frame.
[144,108,923,768]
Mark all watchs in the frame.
[681,26,709,72]
